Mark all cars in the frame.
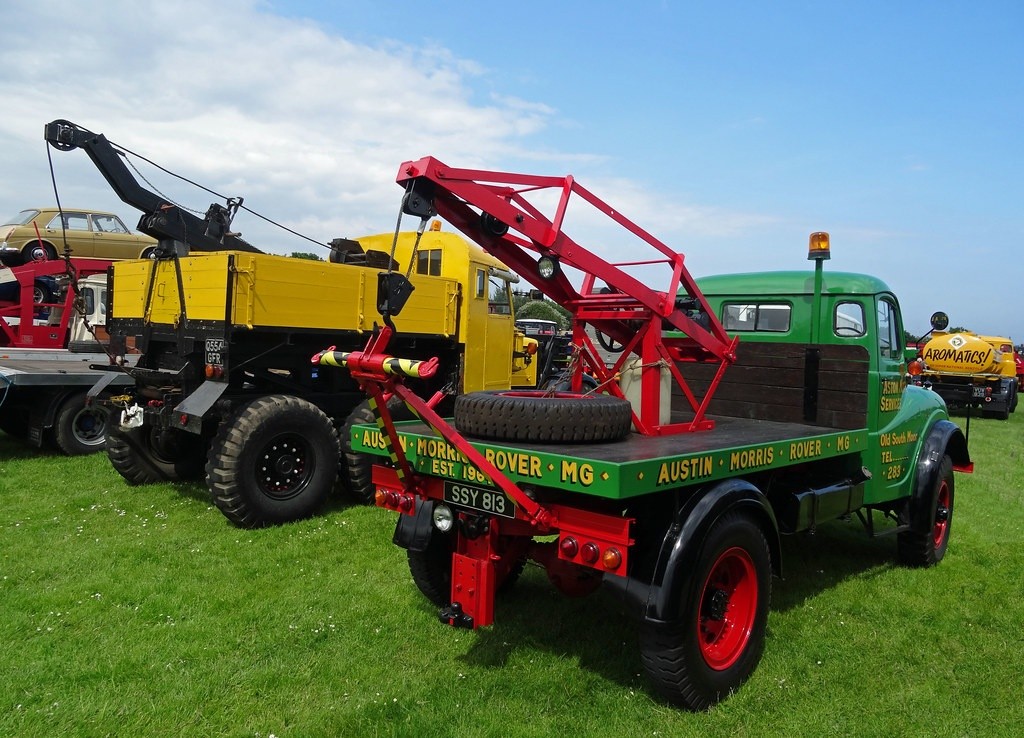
[0,205,164,266]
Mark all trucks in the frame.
[914,332,1019,420]
[308,155,975,711]
[40,119,542,532]
